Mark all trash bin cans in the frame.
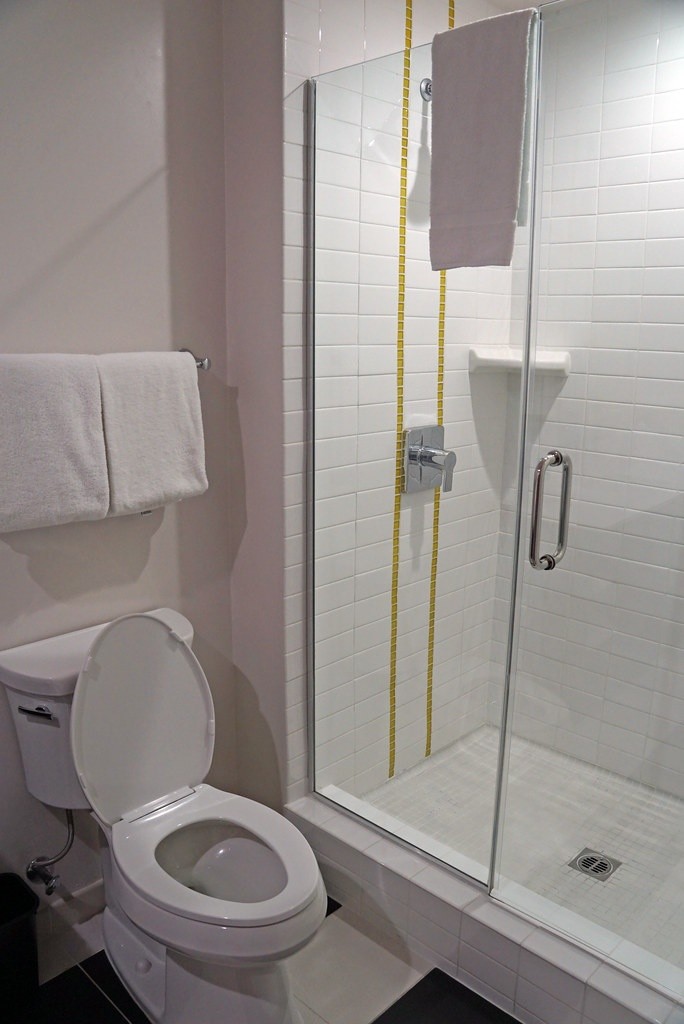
[0,872,40,1024]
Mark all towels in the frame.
[95,351,210,517]
[1,353,109,534]
[427,7,538,273]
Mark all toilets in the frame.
[0,607,327,1024]
